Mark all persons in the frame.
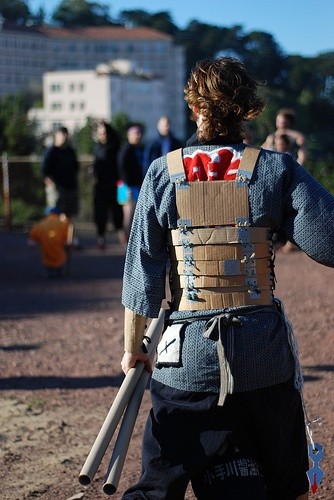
[92,121,127,252]
[120,55,334,500]
[117,123,147,245]
[142,116,185,171]
[40,127,80,249]
[258,109,306,255]
